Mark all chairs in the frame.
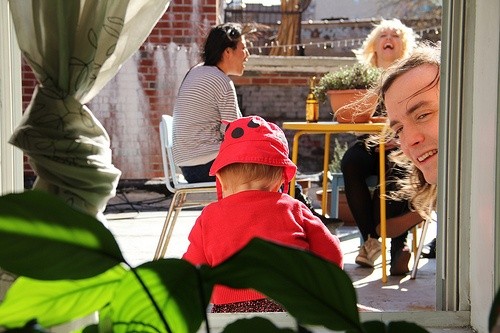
[152,114,319,263]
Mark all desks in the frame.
[282,120,416,283]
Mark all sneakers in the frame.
[355,233,382,267]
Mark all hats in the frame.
[209,116,297,182]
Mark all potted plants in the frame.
[316,138,377,227]
[313,62,386,124]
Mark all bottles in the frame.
[305,77,320,123]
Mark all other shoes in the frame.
[389,246,412,276]
[417,238,437,259]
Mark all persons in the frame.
[173,24,344,232]
[340,19,415,273]
[381,45,441,214]
[183,115,342,313]
